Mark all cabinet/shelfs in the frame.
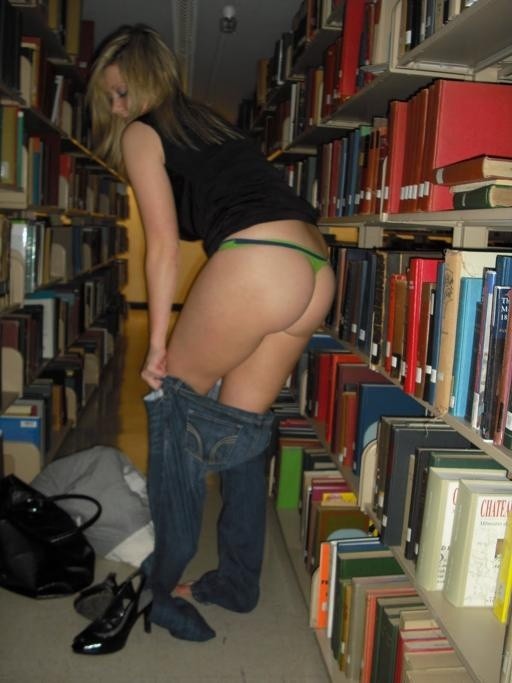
[0,0,128,484]
[270,0,510,680]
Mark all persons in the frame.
[78,21,338,616]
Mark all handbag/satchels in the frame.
[1,474,102,598]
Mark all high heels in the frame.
[72,571,153,655]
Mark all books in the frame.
[232,0,510,682]
[1,0,129,490]
